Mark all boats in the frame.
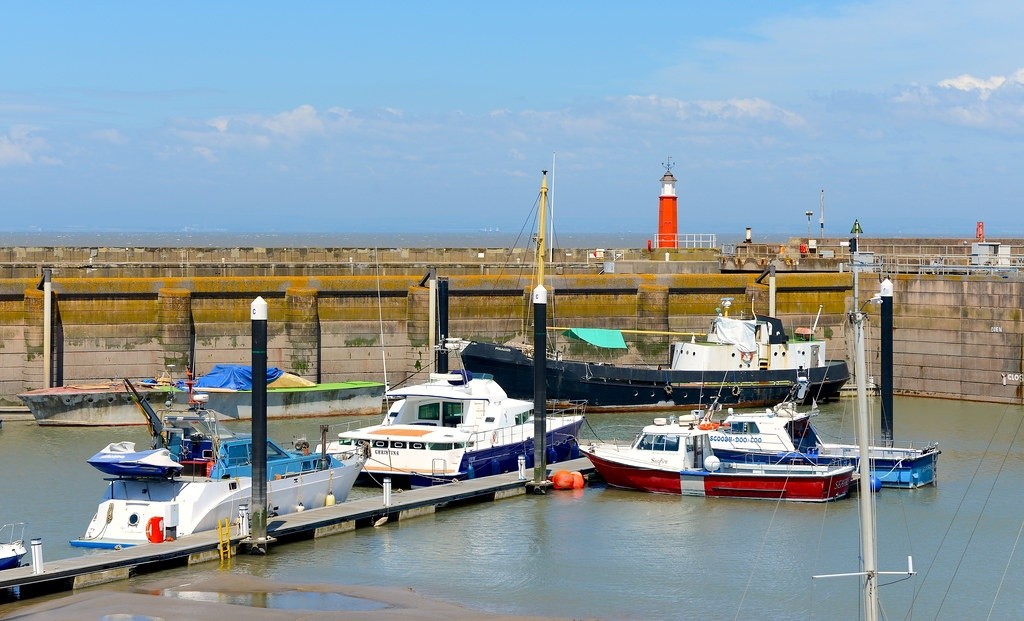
[312,367,588,488]
[63,375,370,555]
[717,401,942,490]
[577,410,855,504]
[430,304,852,403]
[0,522,29,572]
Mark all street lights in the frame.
[806,211,814,238]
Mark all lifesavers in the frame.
[594,251,606,260]
[699,421,730,429]
[741,351,753,361]
